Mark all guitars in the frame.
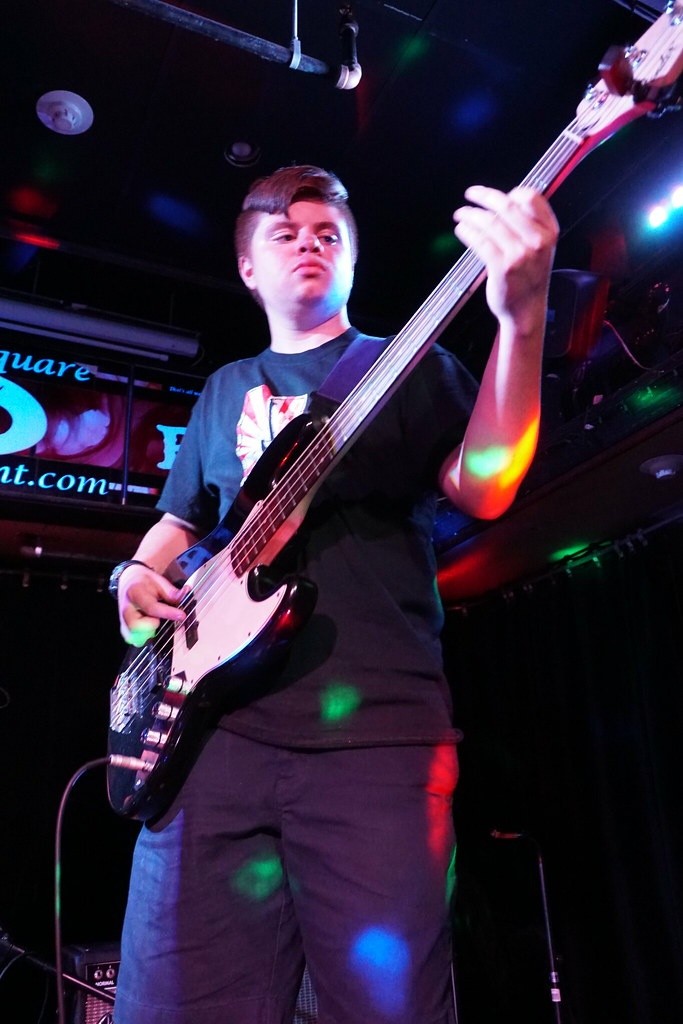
[100,1,681,833]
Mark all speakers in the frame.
[48,942,321,1024]
[542,269,612,370]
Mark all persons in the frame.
[108,163,558,1024]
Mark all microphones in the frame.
[486,828,525,841]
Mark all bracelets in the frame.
[110,561,156,598]
